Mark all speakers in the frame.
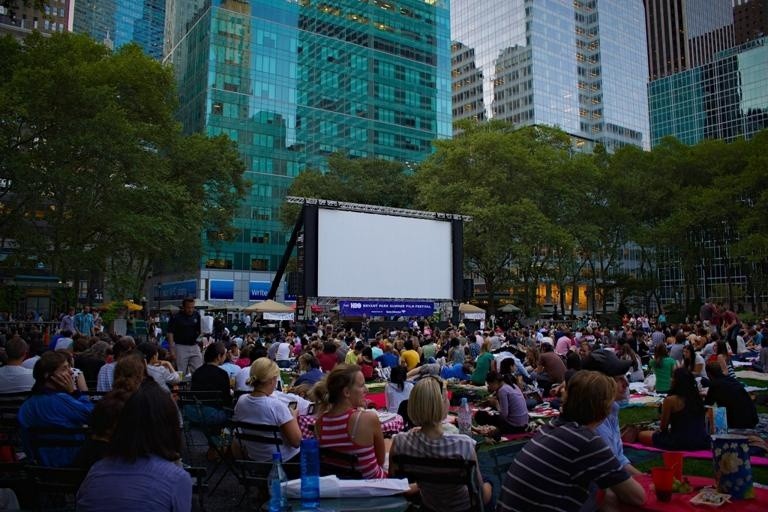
[464,279,473,298]
[289,272,304,295]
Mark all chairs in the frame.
[0,324,768,509]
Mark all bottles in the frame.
[456,397,474,437]
[229,372,236,386]
[265,452,289,512]
[298,439,322,509]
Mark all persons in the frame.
[1,300,768,512]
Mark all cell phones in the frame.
[288,402,297,411]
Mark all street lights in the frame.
[583,289,591,321]
[158,281,162,318]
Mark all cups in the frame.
[662,452,683,481]
[652,466,675,502]
[284,384,289,392]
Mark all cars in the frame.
[543,303,571,321]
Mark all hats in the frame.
[31,351,68,391]
[585,349,632,376]
